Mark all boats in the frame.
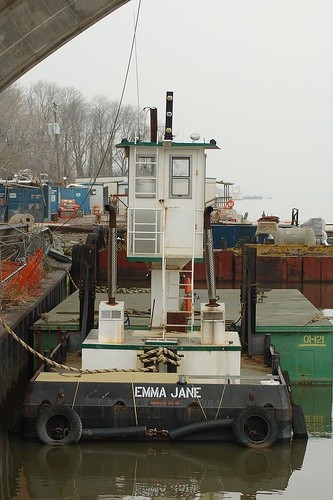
[17,439,308,499]
[20,89,308,449]
